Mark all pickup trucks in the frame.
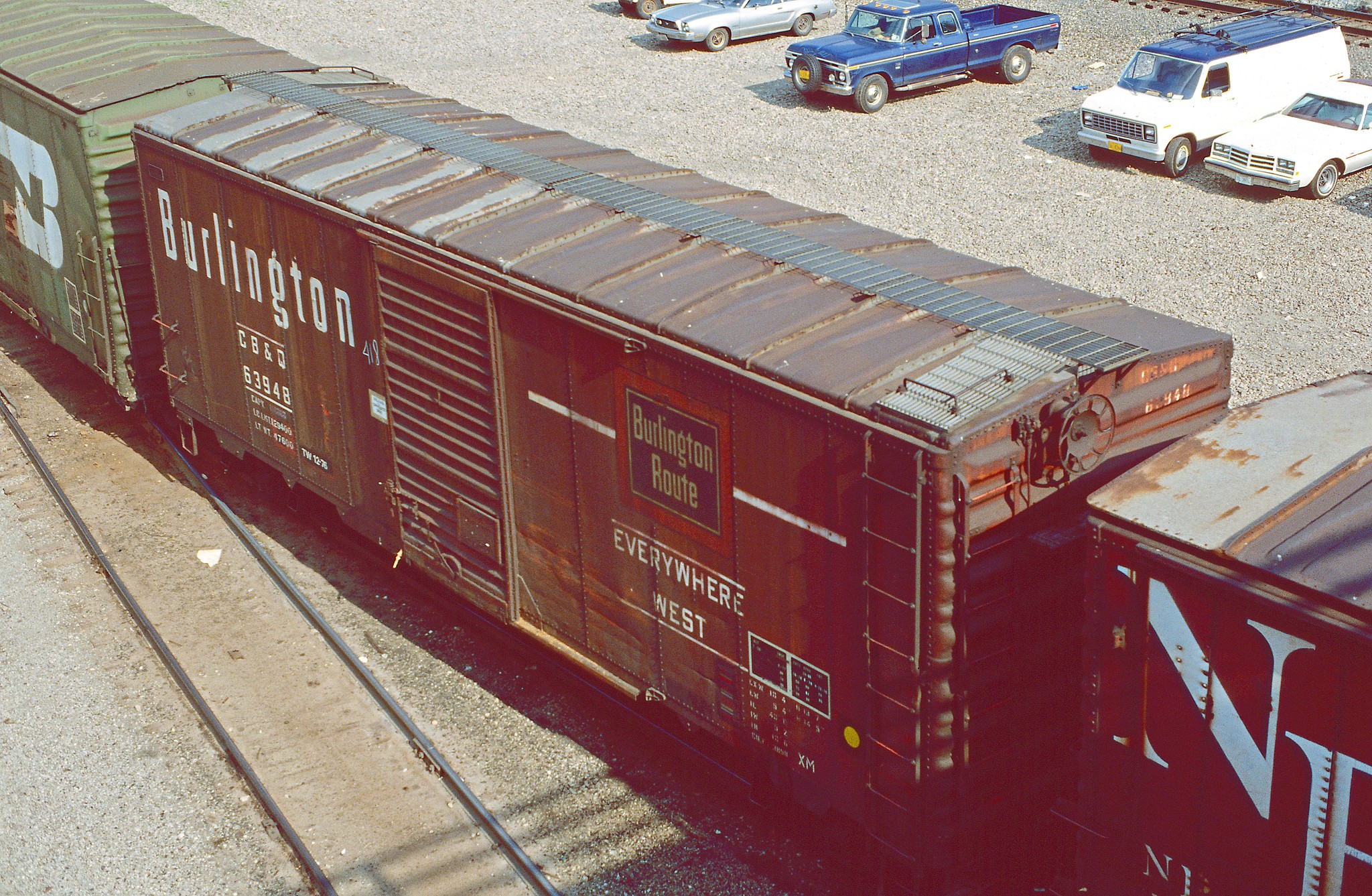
[783,0,1061,115]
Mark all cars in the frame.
[1204,78,1372,200]
[617,0,837,52]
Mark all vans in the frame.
[1076,0,1352,180]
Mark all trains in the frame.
[0,0,1371,896]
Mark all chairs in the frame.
[876,16,887,31]
